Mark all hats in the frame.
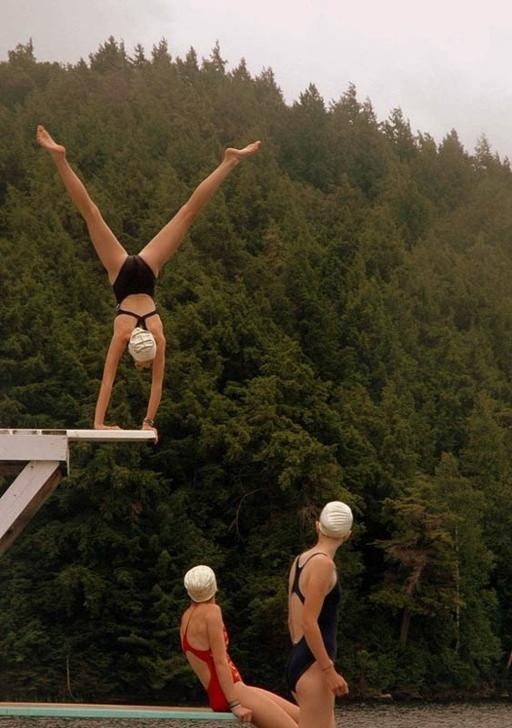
[319,501,353,536]
[128,327,157,362]
[183,564,218,603]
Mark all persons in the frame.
[288,501,353,728]
[37,124,261,445]
[180,564,300,728]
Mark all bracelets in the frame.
[144,417,155,426]
[228,698,241,709]
[322,661,335,671]
